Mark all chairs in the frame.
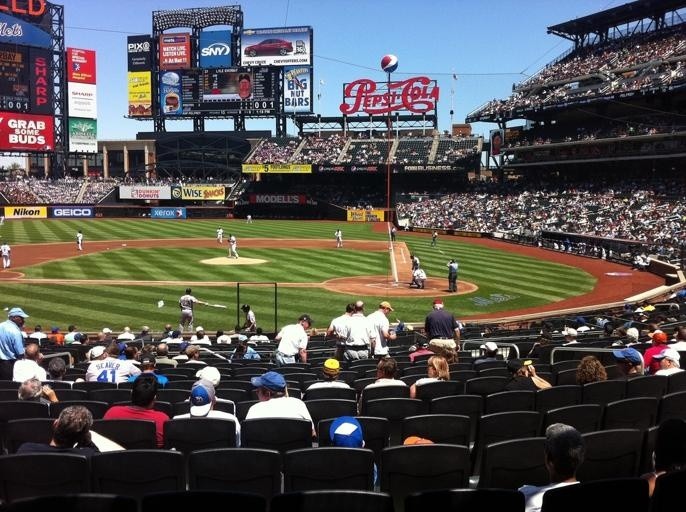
[0,296,686,511]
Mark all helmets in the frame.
[241,304,250,310]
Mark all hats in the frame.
[561,317,681,363]
[189,358,434,447]
[91,346,107,358]
[380,301,394,312]
[103,328,112,334]
[299,314,314,323]
[433,299,443,304]
[480,342,498,353]
[507,358,533,371]
[9,308,29,318]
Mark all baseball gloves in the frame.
[228,239,231,243]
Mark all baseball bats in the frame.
[208,303,227,309]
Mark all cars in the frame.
[245,39,292,57]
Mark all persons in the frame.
[246,130,479,232]
[1,175,252,223]
[391,227,397,241]
[431,231,439,245]
[1,287,465,493]
[227,233,239,259]
[216,225,224,244]
[1,242,11,268]
[518,423,586,511]
[234,73,253,99]
[473,285,685,393]
[479,25,685,272]
[335,229,343,248]
[640,418,684,510]
[76,230,83,250]
[446,259,458,292]
[409,256,427,289]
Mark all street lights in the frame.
[449,74,458,137]
[317,79,324,138]
